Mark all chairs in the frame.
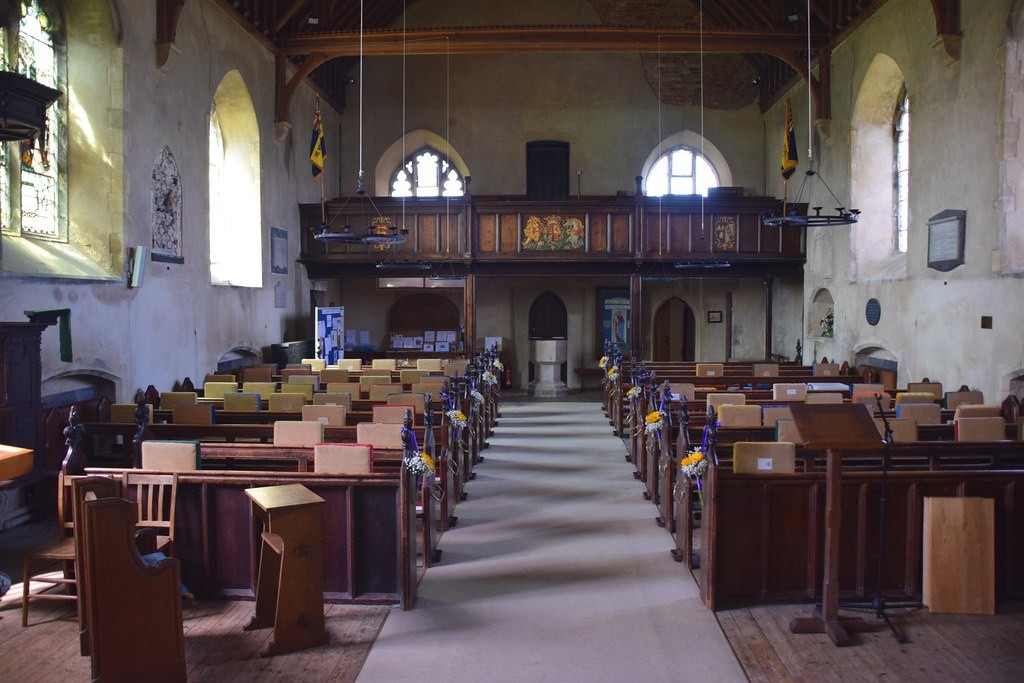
[121,470,180,560]
[19,471,113,627]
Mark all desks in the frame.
[52,407,422,613]
[243,483,332,654]
[699,406,1024,610]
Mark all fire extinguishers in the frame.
[505,361,512,385]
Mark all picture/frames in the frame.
[707,310,723,323]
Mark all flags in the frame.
[308,90,328,181]
[780,97,798,180]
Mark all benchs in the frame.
[602,338,1024,571]
[42,345,503,683]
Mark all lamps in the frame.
[313,0,464,283]
[627,1,861,285]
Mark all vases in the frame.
[825,327,833,338]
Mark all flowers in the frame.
[402,356,707,482]
[819,309,834,330]
